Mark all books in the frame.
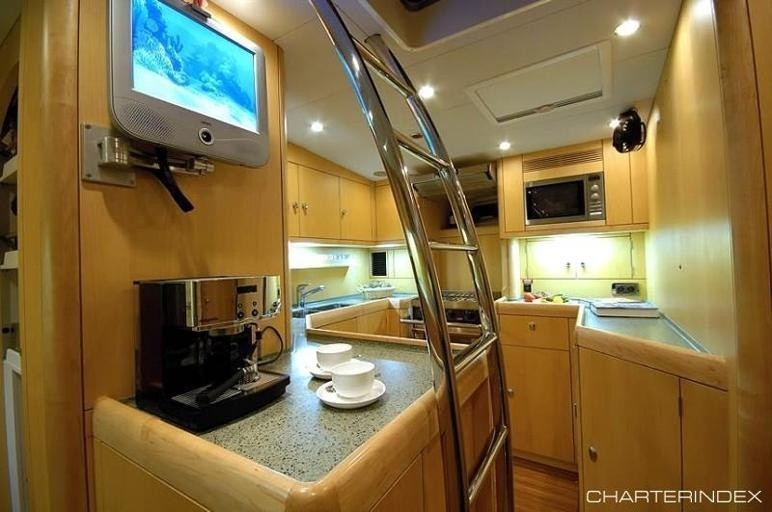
[591,298,662,319]
[579,297,642,309]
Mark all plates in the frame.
[309,359,362,379]
[315,379,387,411]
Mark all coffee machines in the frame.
[132,272,291,438]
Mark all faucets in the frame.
[297,282,329,305]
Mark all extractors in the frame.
[409,161,497,205]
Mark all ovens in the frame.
[409,324,484,345]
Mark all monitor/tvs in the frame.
[107,0,271,169]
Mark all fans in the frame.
[612,108,647,154]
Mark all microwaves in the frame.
[522,170,605,227]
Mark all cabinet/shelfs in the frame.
[341,166,447,246]
[287,141,341,241]
[518,233,646,302]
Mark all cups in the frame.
[314,343,353,373]
[330,360,376,399]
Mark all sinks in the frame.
[306,303,352,310]
[292,309,318,318]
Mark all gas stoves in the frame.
[398,291,503,325]
